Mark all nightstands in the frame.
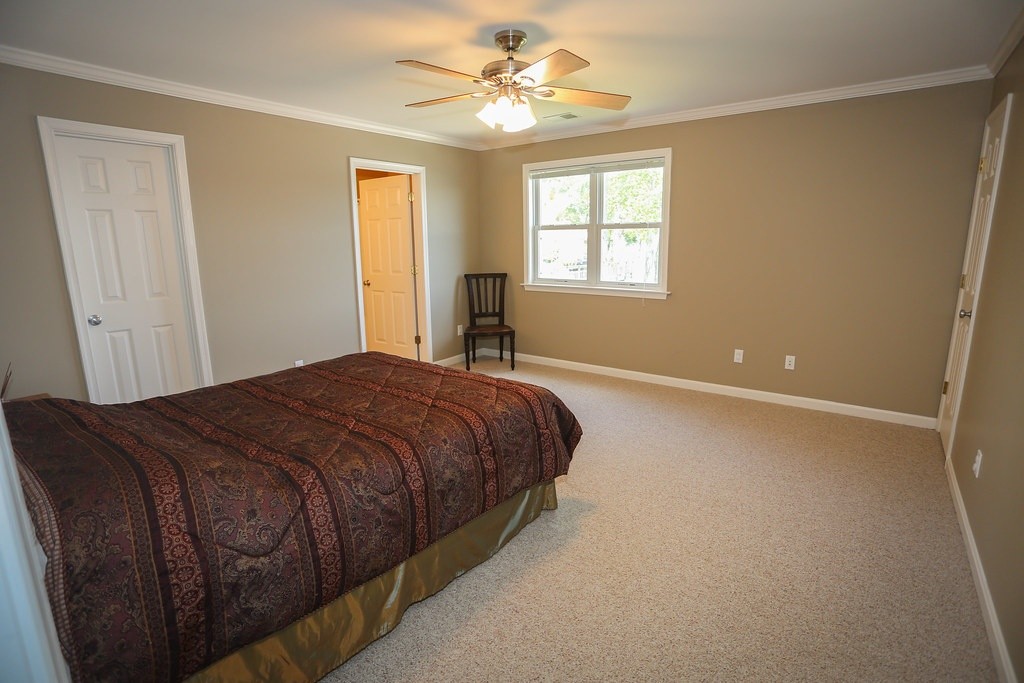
[11,393,51,401]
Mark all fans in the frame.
[394,29,632,133]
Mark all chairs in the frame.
[460,272,515,371]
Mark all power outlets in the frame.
[294,359,304,367]
[971,449,983,480]
[784,354,796,370]
[733,349,744,364]
[0,350,583,683]
[458,325,464,336]
